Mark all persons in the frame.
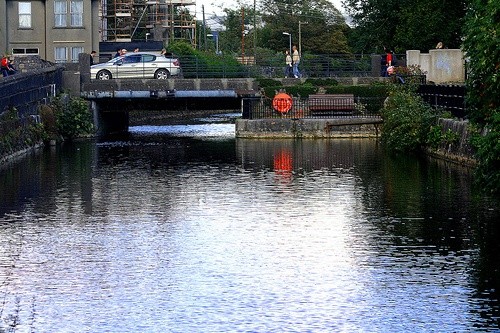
[284,50,295,78]
[129,47,141,63]
[113,48,127,59]
[0,55,19,77]
[381,46,398,77]
[292,46,303,79]
[90,51,96,65]
[161,48,177,59]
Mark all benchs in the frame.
[308,94,354,118]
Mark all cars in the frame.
[90,51,181,80]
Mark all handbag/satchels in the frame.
[387,65,394,74]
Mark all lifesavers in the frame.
[274,98,291,112]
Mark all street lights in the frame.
[207,34,219,55]
[283,32,292,55]
